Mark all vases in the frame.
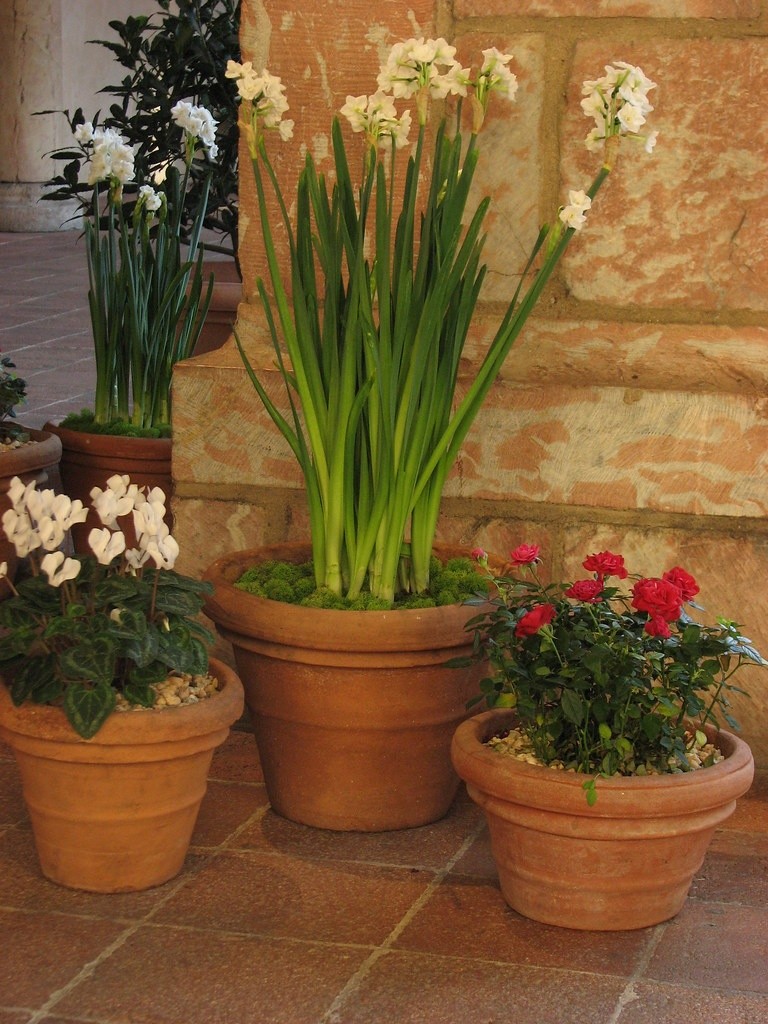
[440,709,755,934]
[0,658,245,893]
[40,420,173,561]
[197,536,526,830]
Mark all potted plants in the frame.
[0,354,76,598]
[39,0,250,356]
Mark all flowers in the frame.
[47,100,218,432]
[203,27,652,582]
[0,470,220,732]
[451,540,766,770]
